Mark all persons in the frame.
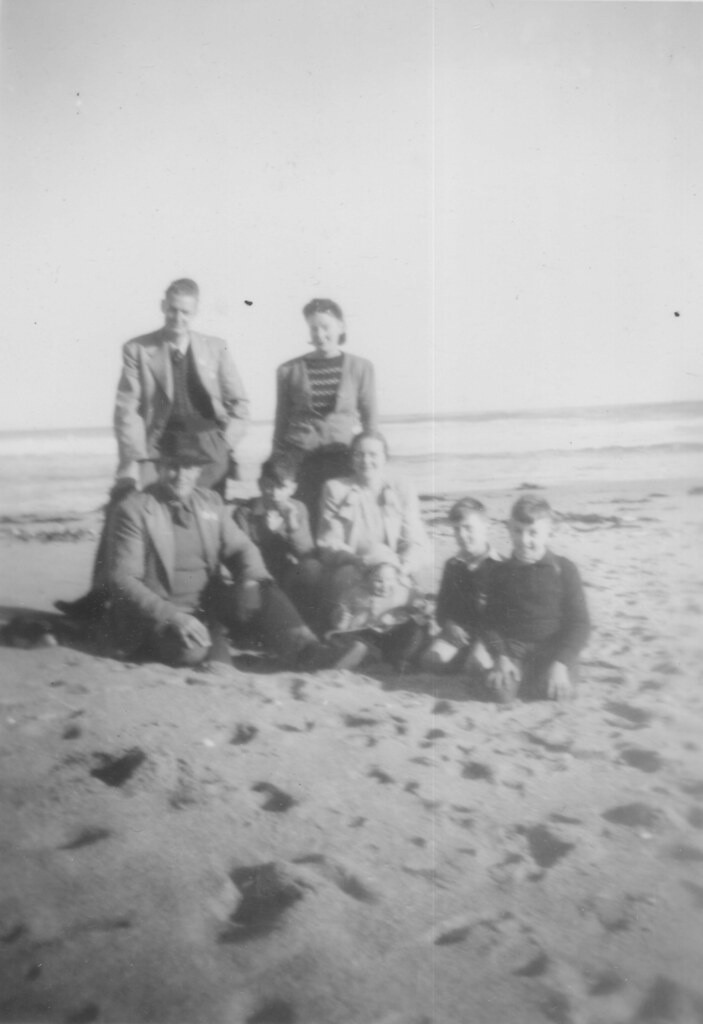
[269,297,385,548]
[50,272,254,617]
[106,428,592,702]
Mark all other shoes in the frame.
[297,639,368,670]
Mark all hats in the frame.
[154,430,216,465]
